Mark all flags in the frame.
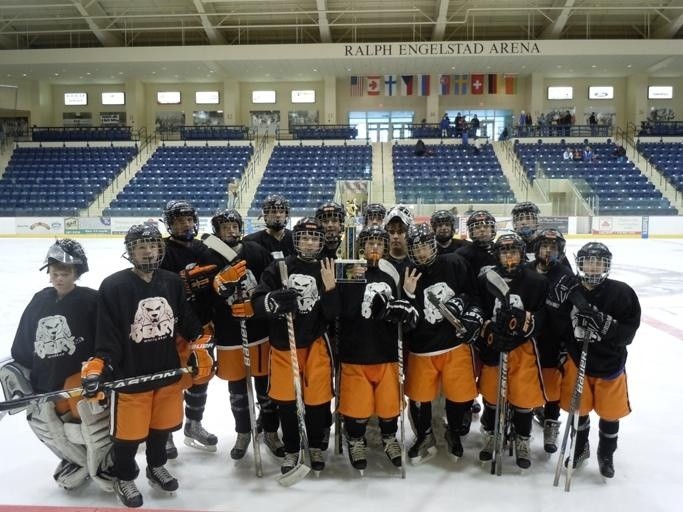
[350,73,522,97]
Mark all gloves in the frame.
[214,258,248,298]
[187,335,217,381]
[268,290,299,317]
[81,359,112,403]
[179,263,218,300]
[370,293,536,366]
[575,307,611,336]
[230,300,254,320]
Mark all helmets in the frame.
[574,242,611,287]
[46,237,89,275]
[211,209,244,246]
[262,195,290,230]
[291,216,326,262]
[125,223,166,271]
[316,201,346,250]
[356,203,567,277]
[163,200,200,242]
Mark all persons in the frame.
[414,109,658,161]
[2,238,116,492]
[156,191,642,482]
[228,176,240,208]
[82,221,204,507]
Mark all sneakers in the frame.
[346,437,368,474]
[146,465,178,491]
[470,398,480,418]
[281,452,299,474]
[542,420,559,453]
[231,429,250,460]
[515,432,532,469]
[408,427,438,466]
[263,430,287,462]
[309,447,325,475]
[478,432,501,462]
[115,480,143,508]
[381,432,403,470]
[534,408,546,426]
[444,430,463,462]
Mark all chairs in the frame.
[0,123,683,216]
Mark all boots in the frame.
[166,432,178,459]
[596,446,614,478]
[185,418,218,445]
[564,441,590,468]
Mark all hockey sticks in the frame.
[378,259,407,479]
[554,291,593,491]
[487,267,512,476]
[202,234,262,478]
[276,261,313,487]
